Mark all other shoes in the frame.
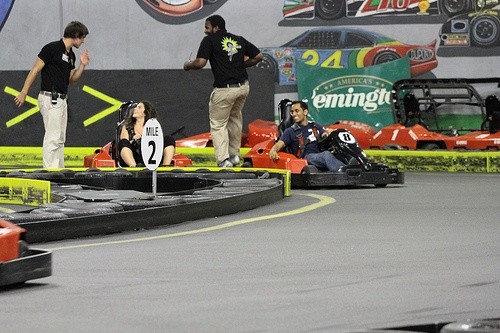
[345,157,357,164]
[229,156,240,164]
[218,159,233,166]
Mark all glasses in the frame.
[289,109,303,114]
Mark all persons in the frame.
[269,102,359,171]
[184,15,264,167]
[118,101,176,167]
[14,21,91,168]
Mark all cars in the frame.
[253,27,438,89]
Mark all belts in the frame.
[217,81,243,88]
[40,91,67,98]
